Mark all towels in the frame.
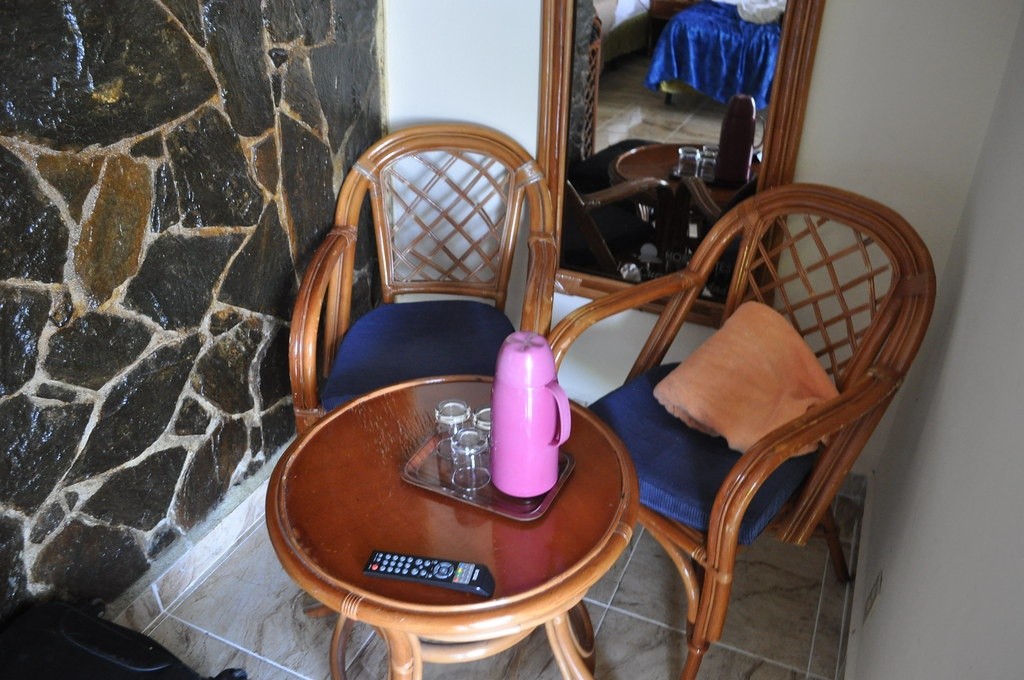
[652,300,842,458]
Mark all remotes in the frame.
[364,550,495,596]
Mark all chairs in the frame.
[289,125,559,437]
[674,173,758,302]
[542,184,936,680]
[565,178,674,284]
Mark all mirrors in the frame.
[536,0,825,328]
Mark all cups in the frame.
[678,146,700,178]
[433,398,473,461]
[702,143,717,160]
[474,406,491,454]
[698,152,717,182]
[451,429,491,492]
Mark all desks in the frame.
[645,0,781,112]
[608,143,760,237]
[265,375,639,680]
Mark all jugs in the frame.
[491,331,571,498]
[716,94,766,183]
